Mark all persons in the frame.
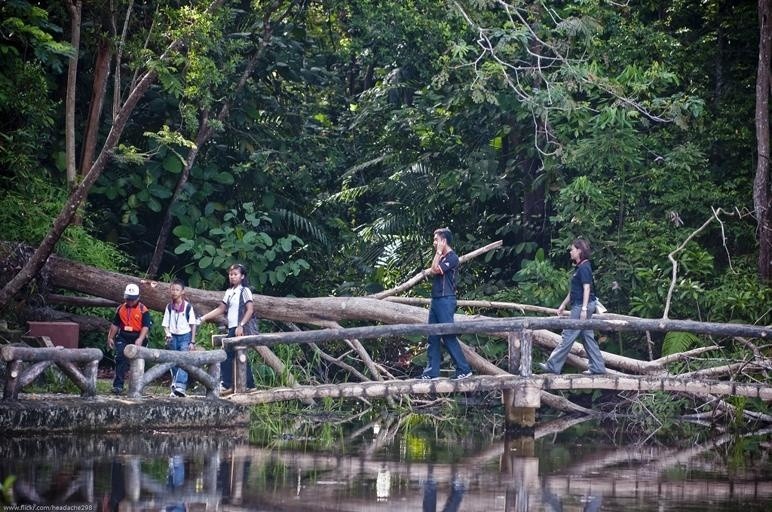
[414,227,472,382]
[106,282,150,396]
[195,264,257,397]
[539,239,607,375]
[161,280,196,397]
[98,454,603,512]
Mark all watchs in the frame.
[436,251,443,256]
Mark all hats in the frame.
[124,284,139,299]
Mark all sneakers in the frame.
[174,388,186,397]
[111,387,122,394]
[450,372,473,381]
[413,374,440,381]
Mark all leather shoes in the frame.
[538,362,553,373]
[584,368,595,374]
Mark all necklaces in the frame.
[173,305,180,329]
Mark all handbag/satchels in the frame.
[595,297,607,314]
[238,286,257,336]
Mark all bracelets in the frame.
[239,321,246,328]
[581,304,588,311]
[190,340,197,344]
